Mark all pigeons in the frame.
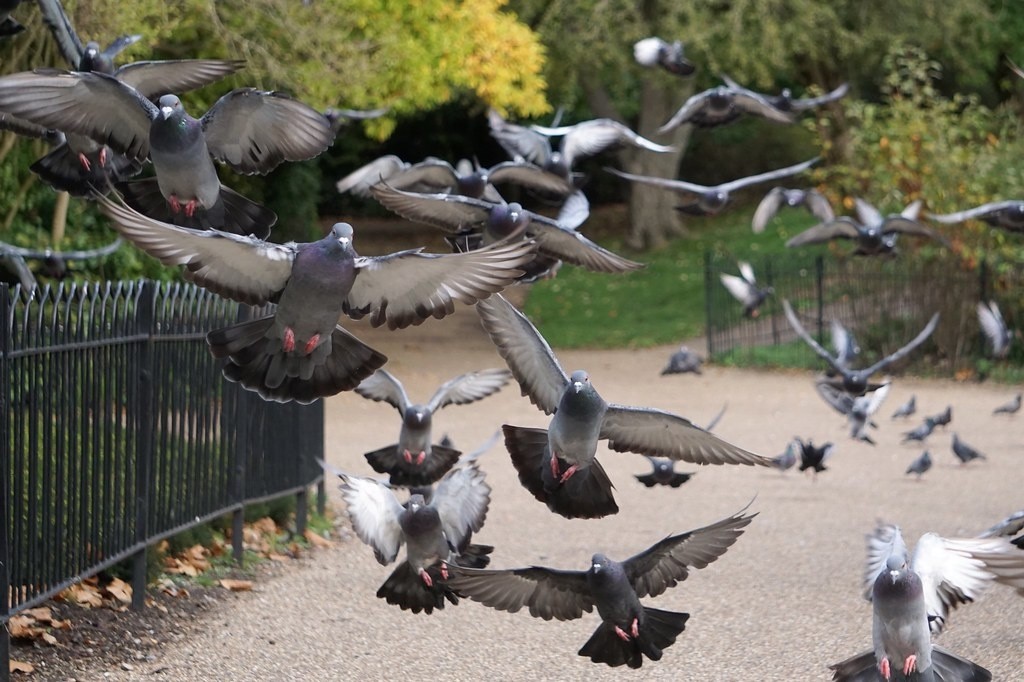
[0,1,1024,682]
